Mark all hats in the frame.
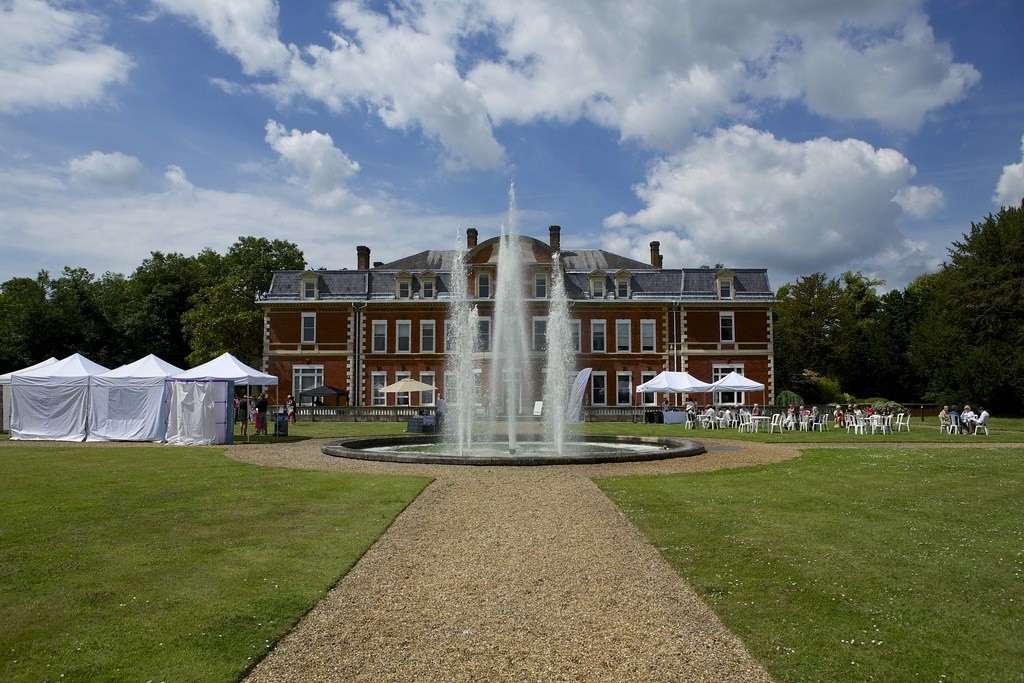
[836,405,842,409]
[853,404,858,408]
[686,405,692,410]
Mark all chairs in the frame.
[938,415,990,436]
[834,409,910,435]
[684,410,829,433]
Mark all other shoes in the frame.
[971,432,975,436]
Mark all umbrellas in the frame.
[378,378,440,406]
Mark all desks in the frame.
[663,412,686,424]
[414,415,437,431]
[801,416,814,430]
[860,417,878,435]
[750,416,771,433]
[697,415,707,424]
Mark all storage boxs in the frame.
[408,418,423,433]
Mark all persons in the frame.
[883,406,893,427]
[787,405,795,413]
[287,394,297,422]
[740,405,749,414]
[702,405,716,426]
[832,403,862,428]
[726,405,733,420]
[751,404,761,416]
[939,405,989,435]
[717,407,725,419]
[864,404,874,423]
[664,400,669,411]
[686,398,697,429]
[233,393,271,437]
[810,406,822,431]
[799,406,810,422]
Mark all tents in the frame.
[165,347,279,446]
[634,370,766,423]
[0,351,187,445]
[298,386,348,422]
